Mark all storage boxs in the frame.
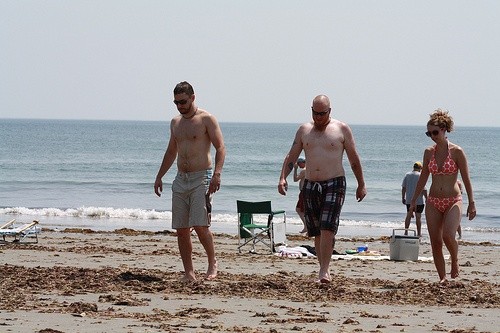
[390,229,420,261]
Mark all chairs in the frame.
[237,200,287,254]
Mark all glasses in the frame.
[424,128,444,138]
[172,97,191,105]
[311,107,330,116]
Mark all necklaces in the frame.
[183,107,198,119]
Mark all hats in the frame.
[296,156,306,164]
[414,161,422,168]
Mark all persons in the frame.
[401,162,428,237]
[278,95,366,283]
[293,158,307,233]
[153,81,227,281]
[409,109,476,284]
[456,181,463,238]
